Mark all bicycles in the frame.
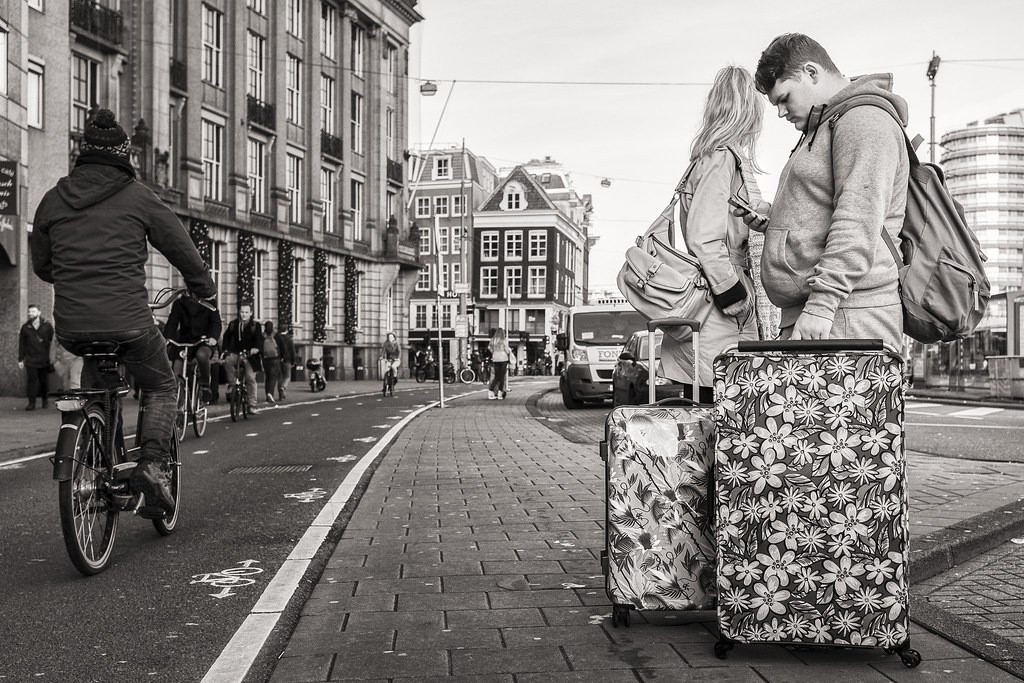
[456,355,475,384]
[47,288,217,576]
[381,358,395,397]
[480,358,491,385]
[169,336,209,443]
[220,349,255,422]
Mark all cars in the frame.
[612,330,684,410]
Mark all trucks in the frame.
[554,297,652,411]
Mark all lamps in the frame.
[421,81,437,96]
[601,178,610,187]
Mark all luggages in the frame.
[711,337,923,668]
[598,320,718,628]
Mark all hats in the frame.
[78,111,131,160]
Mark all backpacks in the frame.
[829,98,991,342]
[263,332,278,359]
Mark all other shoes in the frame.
[497,391,502,400]
[268,393,275,403]
[202,388,211,403]
[26,403,35,411]
[42,400,48,409]
[618,142,743,344]
[394,377,397,383]
[248,406,257,414]
[280,387,286,399]
[488,391,495,399]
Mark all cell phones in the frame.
[728,198,766,224]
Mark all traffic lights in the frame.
[544,336,549,346]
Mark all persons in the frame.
[15,302,567,429]
[29,106,214,512]
[658,65,765,405]
[733,34,907,355]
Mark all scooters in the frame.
[306,356,328,393]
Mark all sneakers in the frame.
[129,458,178,512]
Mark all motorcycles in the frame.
[415,361,455,384]
